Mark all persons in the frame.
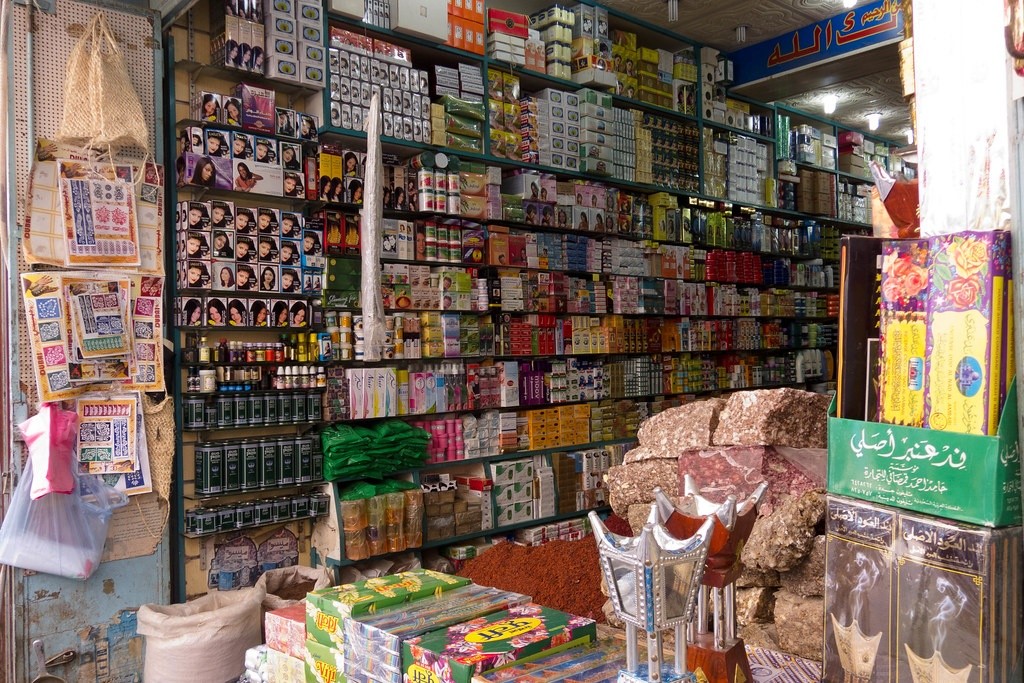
[495,361,505,385]
[177,94,317,199]
[183,201,321,328]
[225,0,266,74]
[382,176,418,211]
[443,276,453,311]
[416,231,427,261]
[614,54,635,99]
[678,92,694,105]
[473,373,481,401]
[526,182,615,234]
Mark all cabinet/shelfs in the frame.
[158,0,910,603]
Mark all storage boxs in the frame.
[173,0,902,600]
[264,568,623,683]
[826,373,1024,529]
